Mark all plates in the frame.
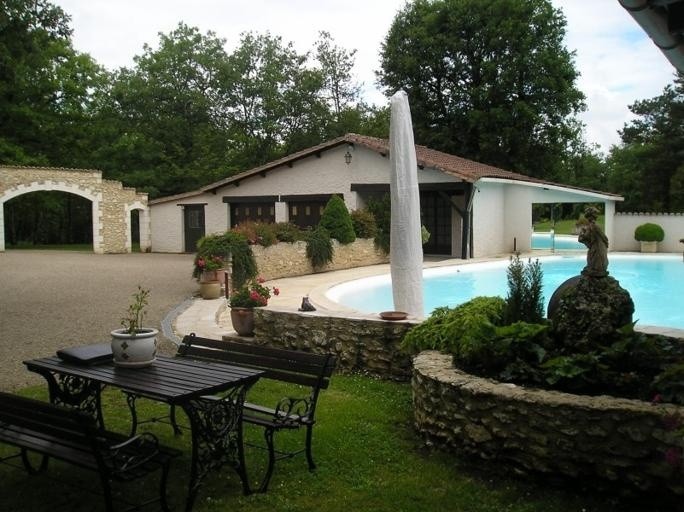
[380,312,408,320]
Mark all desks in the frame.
[20,353,265,510]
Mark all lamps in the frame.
[342,152,353,165]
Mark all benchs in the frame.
[0,390,183,511]
[117,331,338,495]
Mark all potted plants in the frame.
[107,280,159,370]
[634,223,665,254]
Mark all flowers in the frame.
[228,278,279,307]
[197,254,225,271]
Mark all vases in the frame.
[230,307,255,336]
[198,267,226,300]
